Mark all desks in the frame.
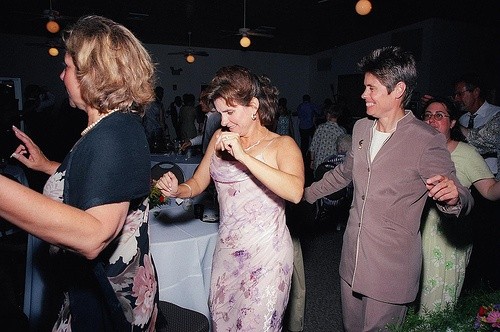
[22,197,218,332]
[0,163,29,237]
[150,151,203,183]
[289,115,317,148]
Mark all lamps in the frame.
[355,0,372,15]
[187,55,194,62]
[240,34,251,47]
[46,17,59,56]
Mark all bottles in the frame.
[174,139,185,155]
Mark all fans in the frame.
[218,0,277,39]
[167,31,209,63]
[8,0,77,49]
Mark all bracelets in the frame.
[180,183,193,198]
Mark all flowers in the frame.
[474,304,500,331]
[150,187,170,208]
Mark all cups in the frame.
[194,204,204,219]
[207,186,217,209]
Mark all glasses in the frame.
[421,112,448,120]
[454,89,470,97]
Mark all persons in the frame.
[279,93,351,235]
[419,101,500,319]
[414,80,500,178]
[154,65,305,332]
[303,45,475,332]
[148,81,222,156]
[0,16,160,332]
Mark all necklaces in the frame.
[244,125,269,152]
[80,108,121,136]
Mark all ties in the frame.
[467,114,478,128]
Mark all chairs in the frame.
[151,160,184,186]
[31,243,209,332]
[313,162,350,237]
